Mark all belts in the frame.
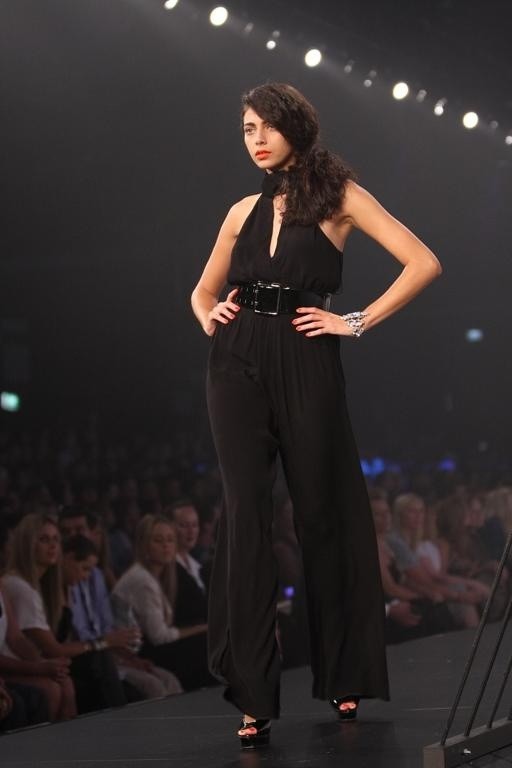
[233,281,329,317]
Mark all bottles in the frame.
[111,602,142,656]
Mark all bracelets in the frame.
[341,310,371,339]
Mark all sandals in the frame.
[330,695,360,721]
[235,716,272,750]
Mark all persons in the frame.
[0,427,512,730]
[1,449,221,729]
[189,84,443,748]
[267,462,512,666]
